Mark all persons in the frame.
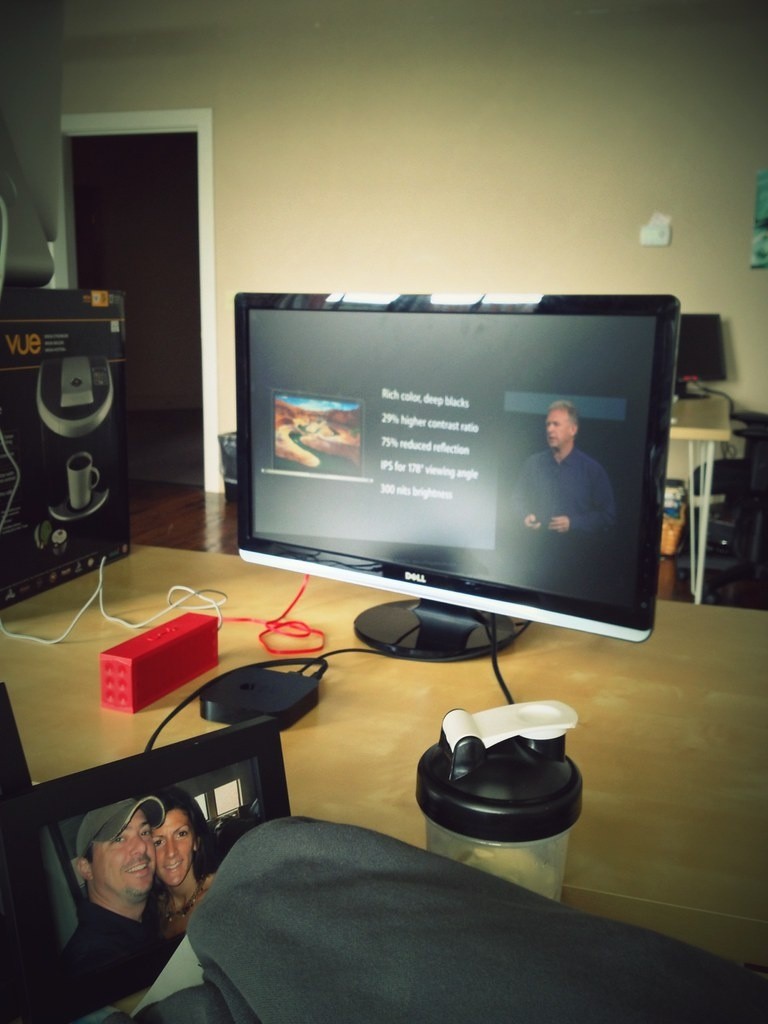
[60,786,224,998]
[511,401,612,599]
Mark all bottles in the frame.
[415,699,584,903]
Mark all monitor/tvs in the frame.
[674,313,726,398]
[234,292,679,665]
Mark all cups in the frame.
[67,452,100,510]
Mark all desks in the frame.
[0,541,768,969]
[671,390,731,604]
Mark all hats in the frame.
[75,796,166,857]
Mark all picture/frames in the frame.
[0,715,291,1024]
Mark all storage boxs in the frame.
[0,284,131,611]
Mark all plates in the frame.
[47,489,109,521]
